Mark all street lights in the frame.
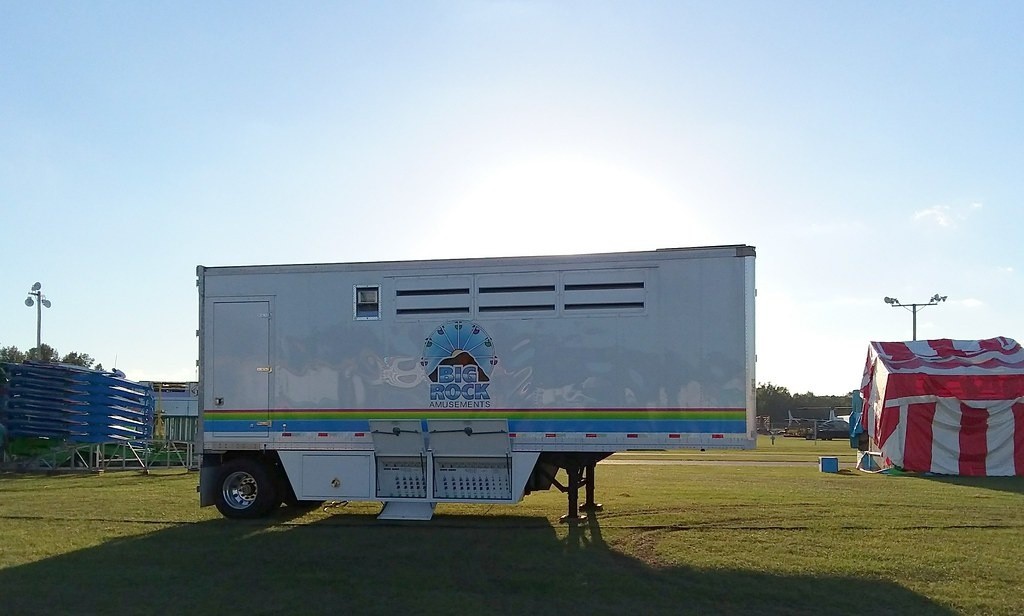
[25,282,52,360]
[884,294,948,342]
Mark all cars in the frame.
[806,420,852,438]
